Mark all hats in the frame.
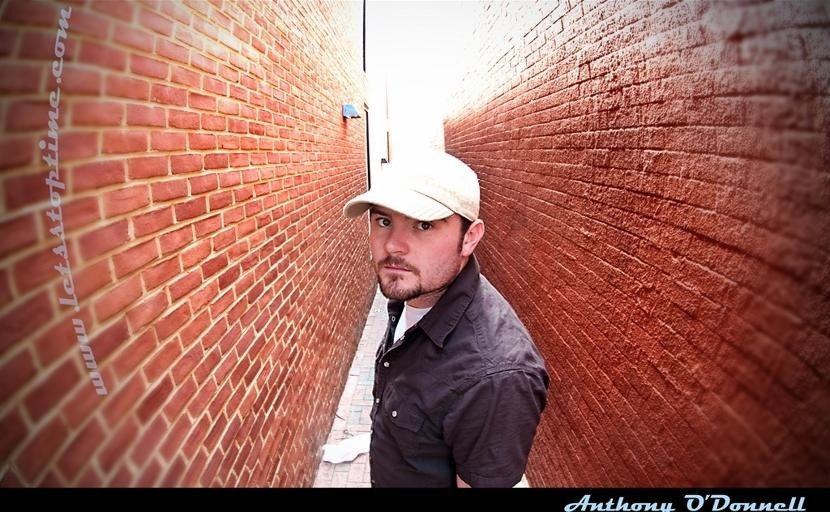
[343,148,480,223]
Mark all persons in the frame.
[342,148,550,488]
